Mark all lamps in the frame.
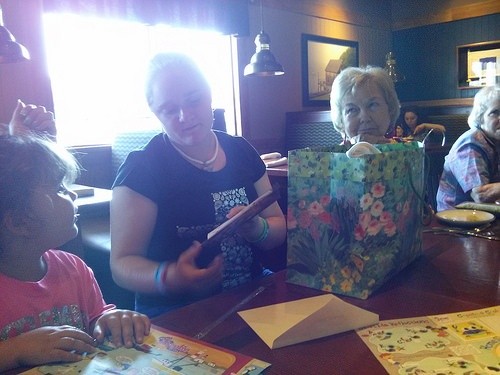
[300,32,360,109]
[382,50,404,89]
[243,0,285,78]
[0,26,32,64]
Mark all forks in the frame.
[423,223,491,233]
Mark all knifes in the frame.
[432,227,499,241]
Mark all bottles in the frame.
[396,126,403,138]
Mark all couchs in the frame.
[284,110,344,158]
[78,131,167,257]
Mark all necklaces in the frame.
[170,130,219,166]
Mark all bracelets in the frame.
[244,216,268,245]
[154,261,170,292]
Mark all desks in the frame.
[70,182,113,213]
[261,153,290,208]
[0,196,500,375]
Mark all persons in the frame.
[395,104,446,143]
[9,98,57,141]
[0,123,151,372]
[327,63,401,144]
[108,53,287,319]
[436,85,500,212]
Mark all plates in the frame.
[402,138,416,141]
[436,209,496,228]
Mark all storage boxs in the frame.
[283,141,427,300]
